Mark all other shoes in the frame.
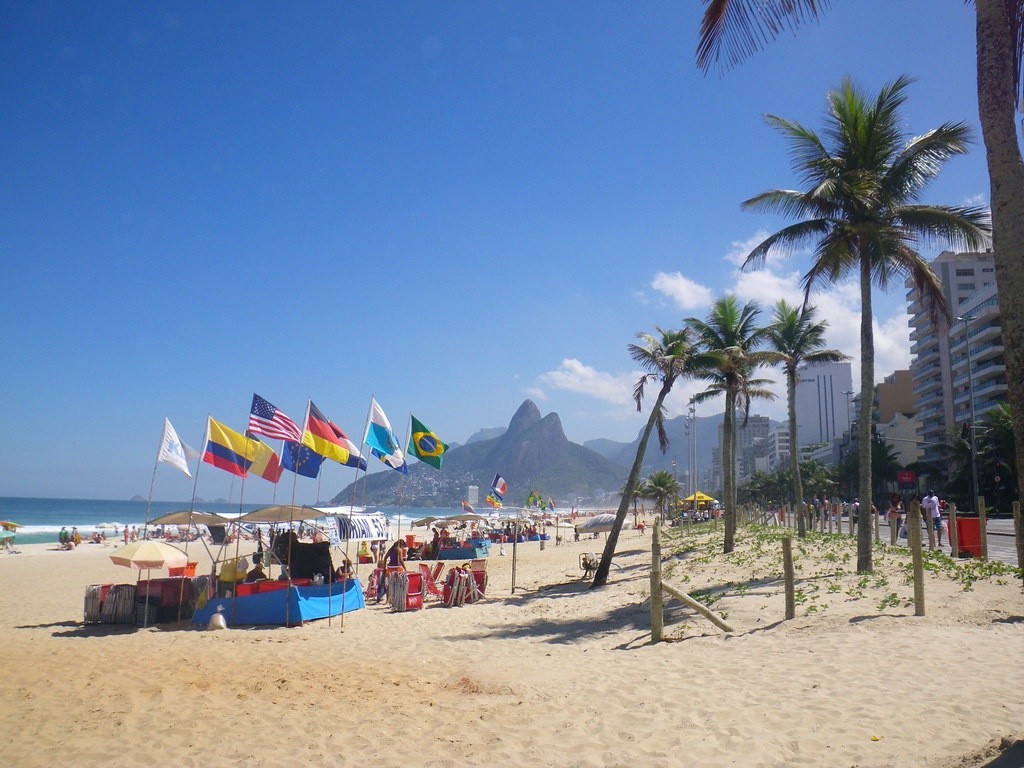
[922,543,927,547]
[938,543,944,547]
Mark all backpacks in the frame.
[852,503,859,515]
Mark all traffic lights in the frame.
[960,422,970,440]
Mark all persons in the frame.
[245,563,267,583]
[885,490,944,547]
[801,495,877,535]
[383,539,407,580]
[359,541,386,563]
[57,524,325,550]
[336,559,353,582]
[431,522,537,559]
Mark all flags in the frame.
[462,500,476,513]
[249,394,303,444]
[485,491,503,509]
[402,416,448,469]
[245,430,284,483]
[363,400,409,478]
[527,490,555,511]
[491,475,507,494]
[157,417,199,478]
[202,416,259,478]
[281,439,321,479]
[301,400,367,470]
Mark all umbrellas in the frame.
[95,522,122,528]
[0,520,22,538]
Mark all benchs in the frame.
[579,553,624,578]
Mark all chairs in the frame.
[366,559,488,612]
[83,576,218,624]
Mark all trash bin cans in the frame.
[948,513,989,558]
[170,562,198,576]
[406,534,417,548]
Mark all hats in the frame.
[255,562,266,570]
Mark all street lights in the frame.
[689,395,697,510]
[685,423,690,496]
[840,390,854,445]
[685,432,689,497]
[686,416,694,497]
[956,313,978,517]
[689,407,696,495]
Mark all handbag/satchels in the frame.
[899,525,908,539]
[940,520,948,535]
